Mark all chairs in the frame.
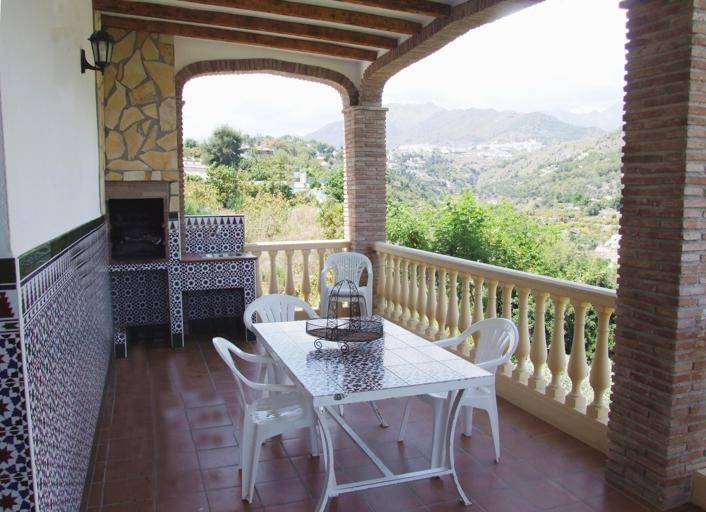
[398,317,519,478]
[243,293,345,416]
[319,251,373,317]
[213,336,328,500]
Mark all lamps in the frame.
[80,25,113,75]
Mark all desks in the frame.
[251,315,493,511]
